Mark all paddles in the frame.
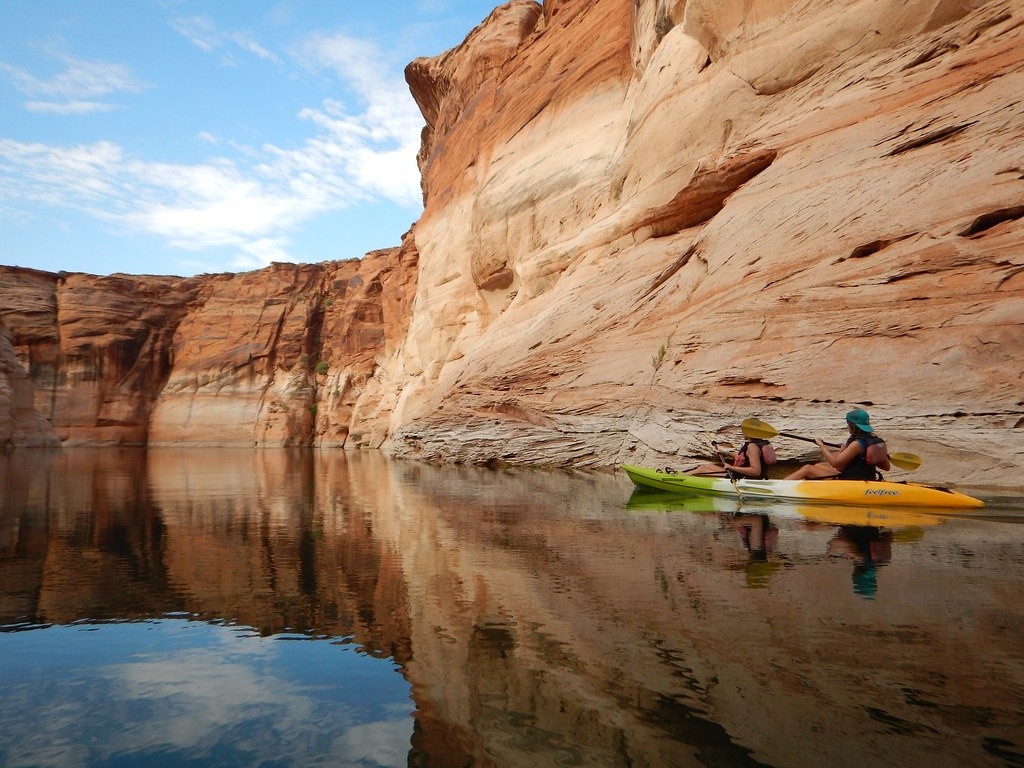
[741,417,922,472]
[710,440,747,517]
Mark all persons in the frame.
[827,525,893,601]
[717,512,781,589]
[783,409,891,480]
[656,418,777,480]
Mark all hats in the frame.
[846,409,873,433]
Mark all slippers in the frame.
[666,466,676,475]
[656,468,664,474]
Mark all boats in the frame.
[619,463,986,509]
